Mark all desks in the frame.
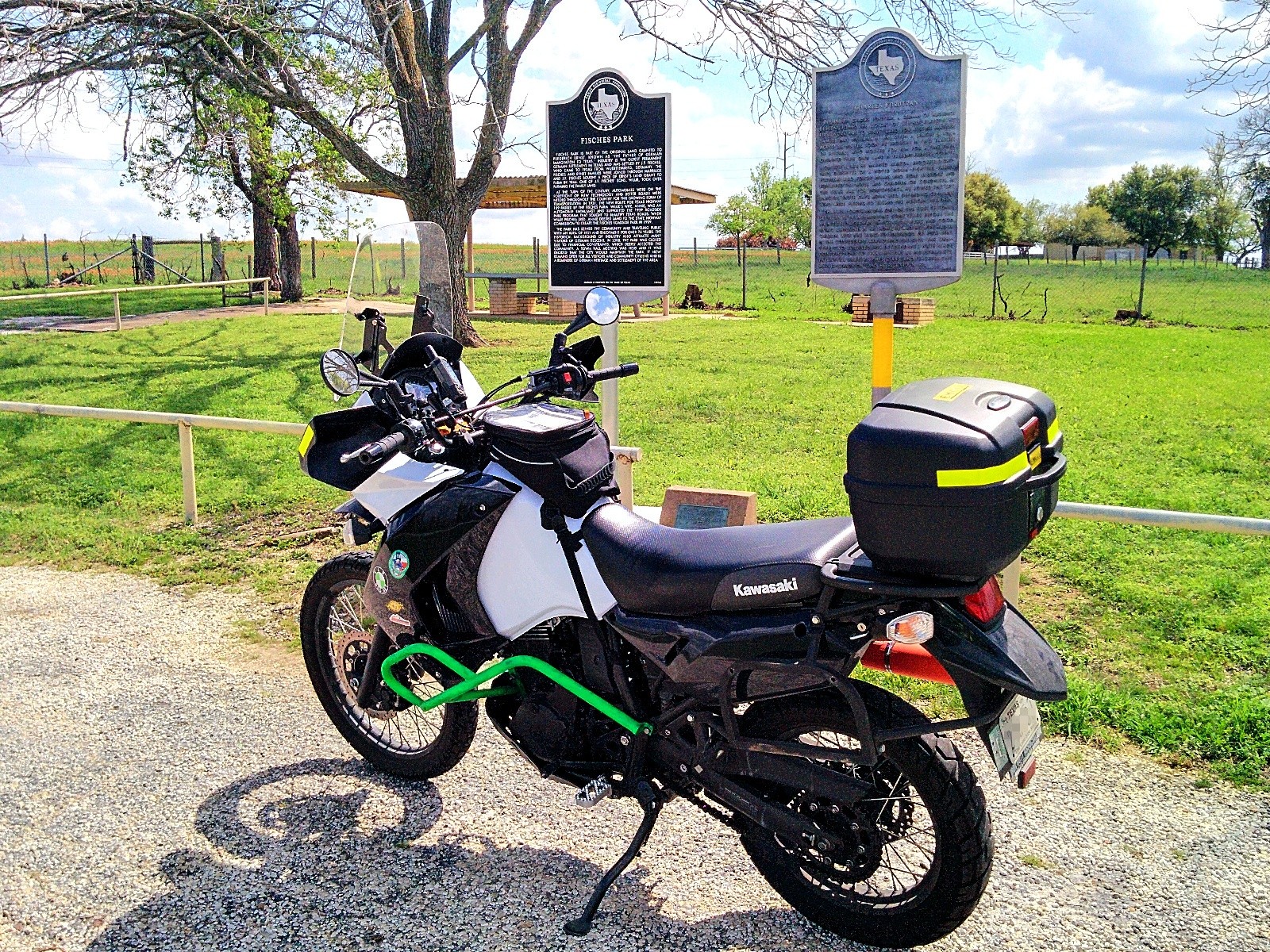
[465,273,577,315]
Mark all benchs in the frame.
[516,292,584,315]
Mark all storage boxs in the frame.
[844,376,1066,575]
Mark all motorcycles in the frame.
[294,221,1069,952]
